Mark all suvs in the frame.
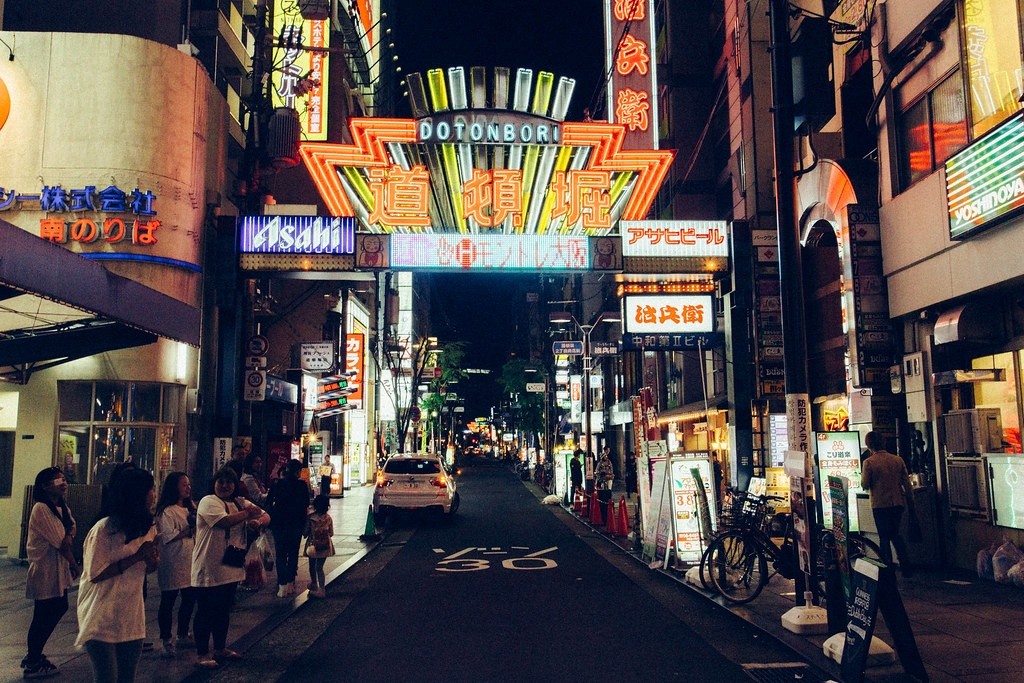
[372,453,459,527]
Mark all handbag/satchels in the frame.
[220,545,247,569]
[240,530,275,589]
[904,517,923,545]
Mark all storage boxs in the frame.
[945,456,991,522]
[943,407,1003,455]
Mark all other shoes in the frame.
[143,642,154,648]
[20,654,50,668]
[901,567,914,582]
[288,582,296,593]
[23,662,58,679]
[307,582,318,591]
[175,632,195,644]
[278,584,289,597]
[313,588,326,597]
[161,640,175,653]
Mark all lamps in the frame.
[366,81,405,108]
[343,12,387,44]
[352,42,394,73]
[384,91,408,118]
[357,55,398,85]
[362,67,401,95]
[346,29,391,58]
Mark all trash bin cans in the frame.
[597,490,612,525]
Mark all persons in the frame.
[155,472,196,658]
[76,462,160,683]
[191,467,271,669]
[20,467,75,678]
[226,444,336,601]
[861,431,916,579]
[570,444,636,506]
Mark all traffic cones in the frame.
[616,500,629,538]
[571,486,582,512]
[588,493,605,526]
[579,489,589,517]
[604,500,618,534]
[620,495,632,529]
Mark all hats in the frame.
[599,452,606,459]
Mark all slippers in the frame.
[194,655,216,665]
[216,648,238,657]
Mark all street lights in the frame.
[388,345,444,453]
[548,310,626,495]
[525,366,550,462]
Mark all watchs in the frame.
[67,531,73,541]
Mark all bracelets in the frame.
[117,559,123,572]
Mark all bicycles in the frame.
[698,482,885,604]
[514,459,553,492]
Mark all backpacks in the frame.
[313,526,330,552]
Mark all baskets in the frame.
[716,499,764,529]
